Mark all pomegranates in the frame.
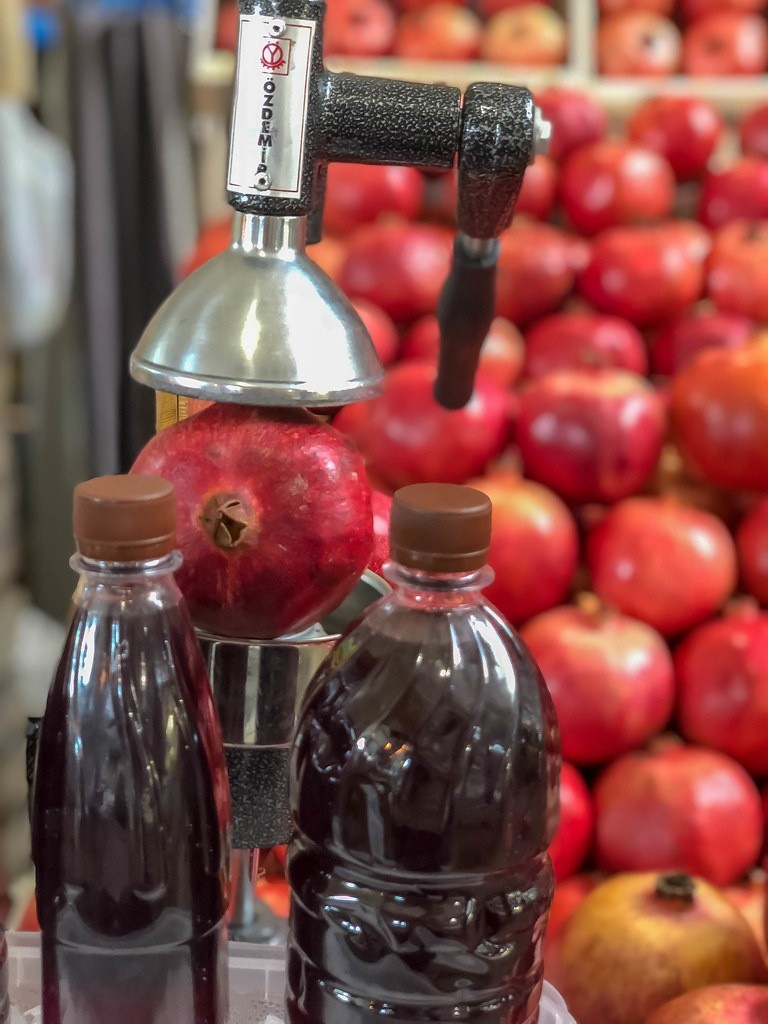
[124,0,768,1024]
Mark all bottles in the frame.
[285,480,559,1024]
[29,475,236,1024]
